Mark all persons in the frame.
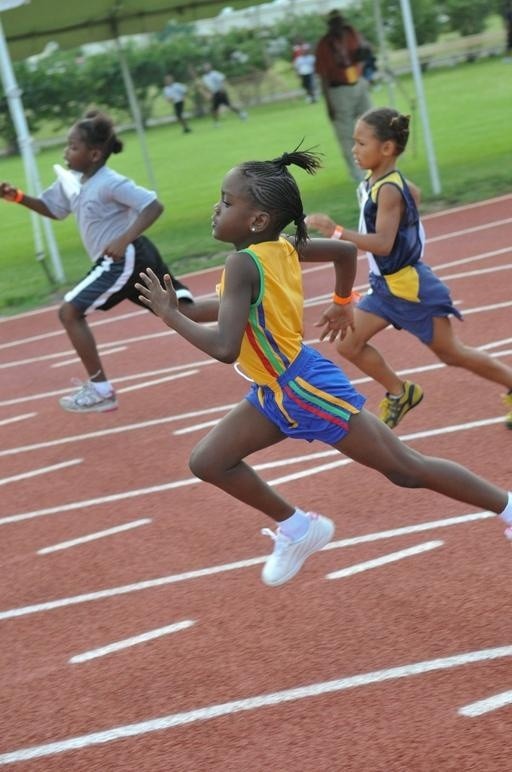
[134,139,512,589]
[166,40,316,133]
[305,106,511,433]
[315,9,381,184]
[0,105,219,410]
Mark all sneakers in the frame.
[59,383,119,414]
[504,388,512,431]
[380,380,424,431]
[261,510,337,588]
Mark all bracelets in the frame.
[334,291,361,305]
[329,225,344,240]
[14,188,23,204]
